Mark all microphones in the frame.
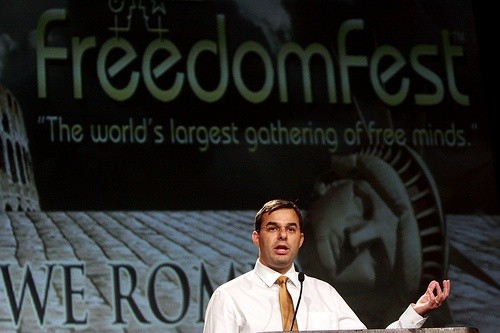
[289,272,306,331]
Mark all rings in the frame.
[434,296,442,305]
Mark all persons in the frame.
[202,199,451,333]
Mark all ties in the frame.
[275,275,298,333]
